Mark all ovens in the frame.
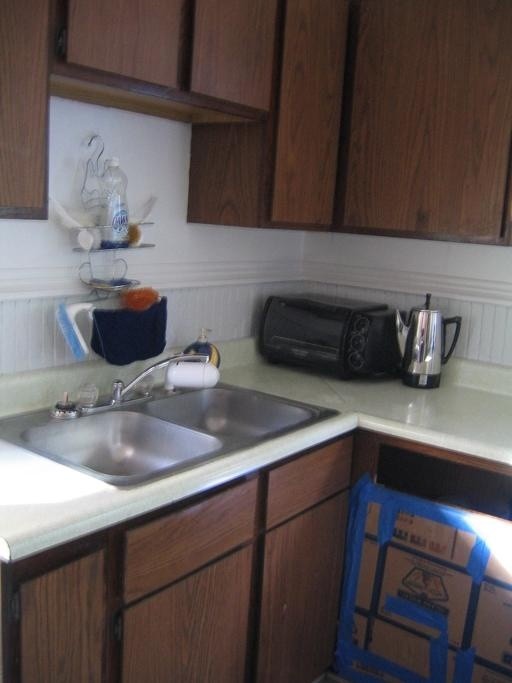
[258,293,397,379]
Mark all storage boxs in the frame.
[338,479,512,683]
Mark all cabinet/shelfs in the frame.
[0,540,113,683]
[52,1,280,126]
[116,427,356,683]
[71,133,155,302]
[184,0,352,232]
[336,1,511,247]
[1,1,50,221]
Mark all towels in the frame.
[90,296,168,367]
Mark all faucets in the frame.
[110,355,209,405]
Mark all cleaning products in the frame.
[183,327,220,368]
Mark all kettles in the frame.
[398,292,461,388]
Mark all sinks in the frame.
[131,381,340,451]
[0,396,227,491]
